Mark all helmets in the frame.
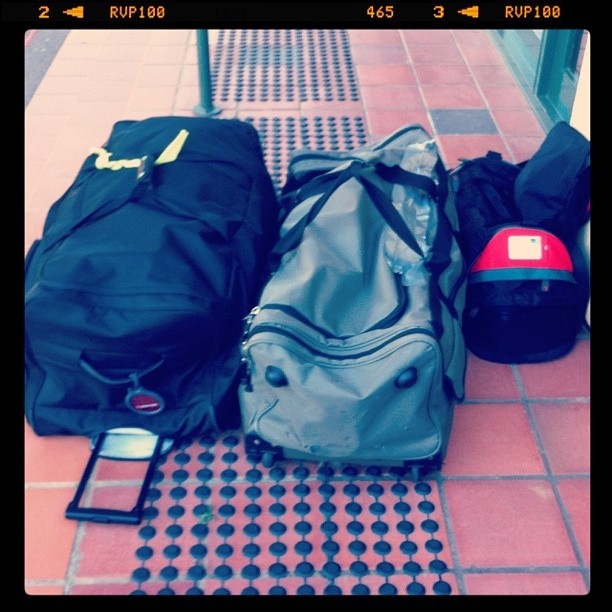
[464,224,577,366]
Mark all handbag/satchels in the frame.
[235,122,468,482]
[23,115,278,526]
[513,121,592,242]
[454,149,527,263]
